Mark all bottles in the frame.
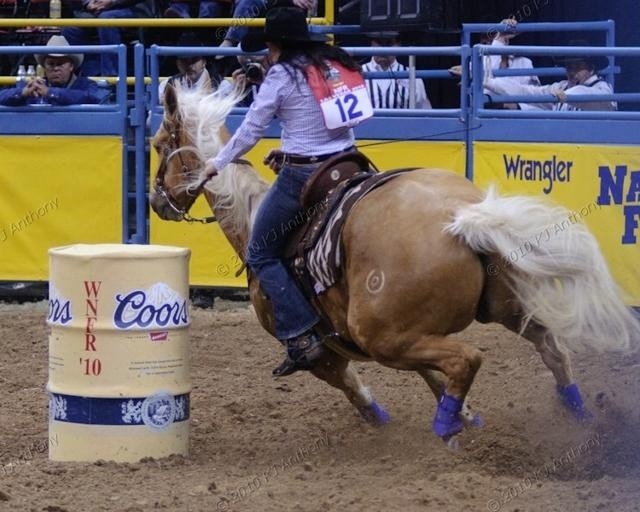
[49,0,61,17]
[17,64,45,76]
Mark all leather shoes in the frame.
[273,332,324,376]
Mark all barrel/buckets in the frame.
[46,242,192,465]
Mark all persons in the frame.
[204,3,355,377]
[1,0,640,114]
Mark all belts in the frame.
[285,145,357,165]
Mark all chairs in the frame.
[0,0,444,94]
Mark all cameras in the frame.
[244,60,262,81]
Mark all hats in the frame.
[552,40,609,69]
[240,7,331,52]
[33,35,84,69]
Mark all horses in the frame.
[150,68,640,455]
